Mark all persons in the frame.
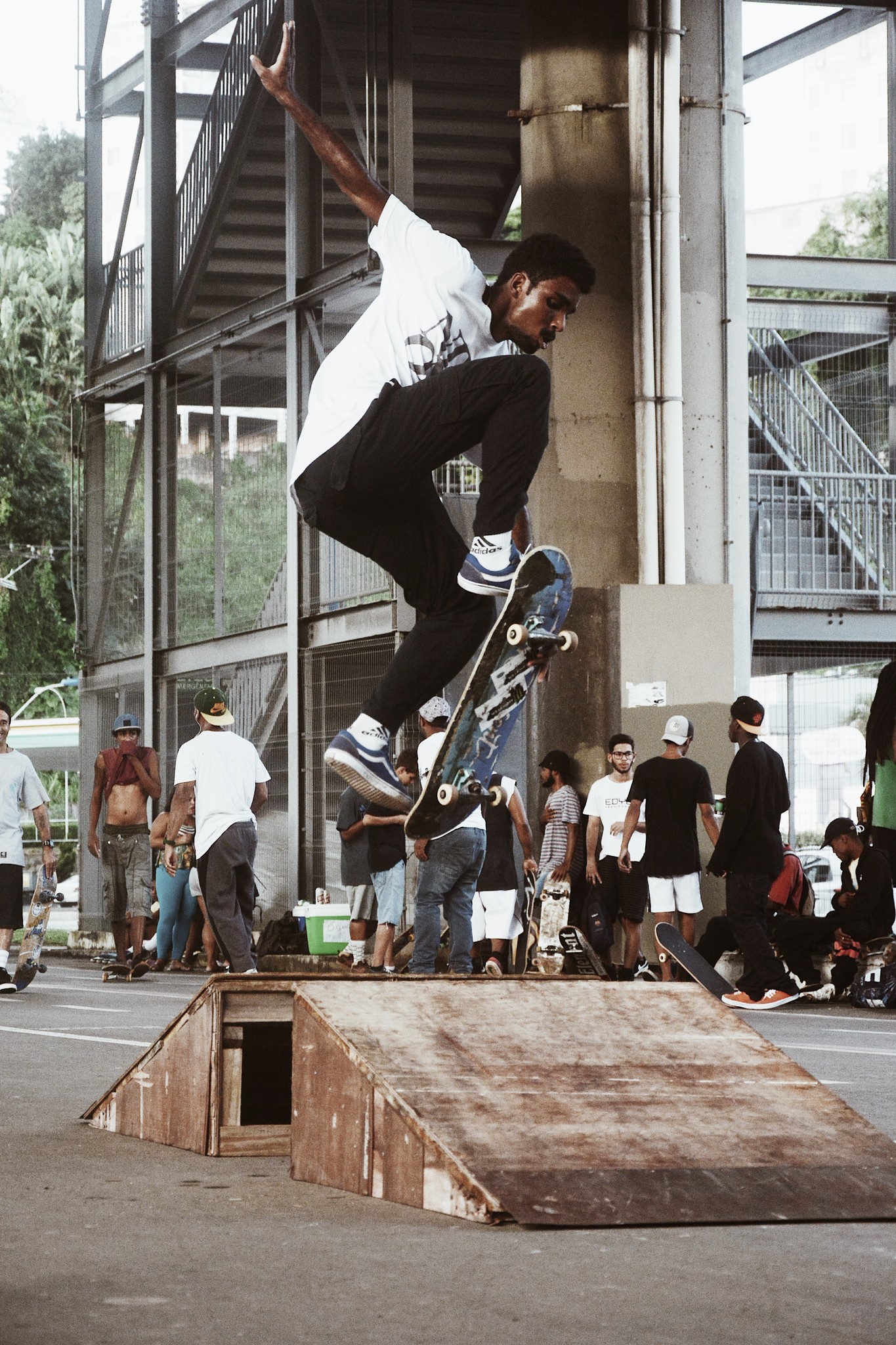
[415,695,488,974]
[776,816,895,1004]
[467,769,538,976]
[864,660,896,886]
[363,748,420,975]
[617,715,733,984]
[679,831,810,984]
[121,782,230,974]
[526,750,585,975]
[336,785,378,974]
[88,713,163,977]
[706,696,801,1011]
[244,16,590,813]
[0,700,58,994]
[165,687,272,976]
[581,733,652,983]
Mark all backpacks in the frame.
[782,850,817,916]
[256,912,307,956]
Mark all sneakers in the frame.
[753,990,800,1010]
[722,991,757,1009]
[457,541,529,597]
[326,729,420,811]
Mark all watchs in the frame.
[41,838,56,848]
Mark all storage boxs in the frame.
[293,903,352,952]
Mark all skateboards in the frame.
[402,544,581,842]
[12,863,65,991]
[559,926,611,982]
[536,869,572,975]
[101,962,132,983]
[514,867,539,975]
[654,922,741,1009]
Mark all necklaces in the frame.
[6,744,10,753]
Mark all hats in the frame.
[114,715,141,734]
[660,715,695,746]
[539,751,572,776]
[730,695,769,735]
[819,818,858,850]
[419,695,453,724]
[194,687,235,727]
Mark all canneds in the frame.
[315,887,330,904]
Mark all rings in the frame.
[841,937,844,940]
[588,876,591,878]
[165,865,167,868]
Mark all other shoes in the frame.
[205,968,224,974]
[225,967,257,974]
[602,962,617,981]
[336,951,354,966]
[0,966,18,994]
[170,966,192,972]
[619,970,634,981]
[633,956,649,976]
[351,960,370,973]
[182,959,201,971]
[150,964,164,972]
[643,970,661,982]
[473,962,486,973]
[382,966,400,974]
[368,964,382,973]
[486,957,505,975]
[131,958,150,978]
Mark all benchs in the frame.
[716,952,884,984]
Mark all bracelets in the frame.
[162,835,176,846]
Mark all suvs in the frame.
[795,846,853,918]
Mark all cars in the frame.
[53,873,80,908]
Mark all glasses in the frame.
[611,751,637,760]
